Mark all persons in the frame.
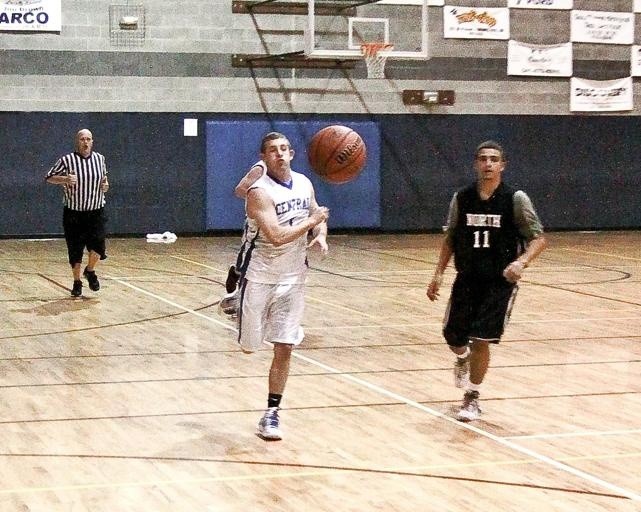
[427,140,548,422]
[42,128,111,297]
[235,132,332,442]
[223,159,269,296]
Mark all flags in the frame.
[376,0,446,8]
[0,0,63,32]
[507,1,575,10]
[569,74,635,112]
[507,38,575,80]
[443,4,511,41]
[630,44,641,78]
[570,8,635,45]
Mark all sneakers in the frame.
[220,291,240,308]
[257,416,283,440]
[71,280,83,295]
[226,264,240,293]
[83,266,100,291]
[457,391,480,421]
[454,355,471,388]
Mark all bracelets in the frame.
[516,255,531,270]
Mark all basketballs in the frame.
[309,125,366,183]
